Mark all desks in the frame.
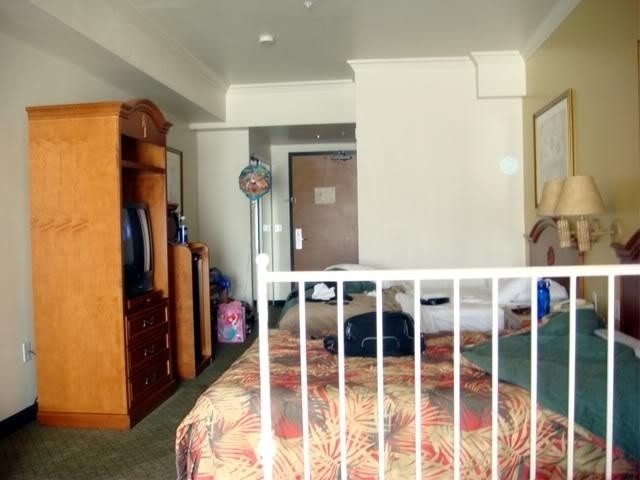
[211,285,227,344]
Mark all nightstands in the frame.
[499,301,532,337]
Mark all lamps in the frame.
[554,176,623,253]
[535,178,601,248]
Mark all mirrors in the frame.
[167,146,184,242]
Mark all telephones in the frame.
[553,298,594,313]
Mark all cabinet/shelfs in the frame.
[25,99,173,432]
[168,243,213,381]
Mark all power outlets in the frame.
[23,343,31,362]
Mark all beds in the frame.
[276,216,584,338]
[174,228,639,480]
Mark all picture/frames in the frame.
[532,88,576,210]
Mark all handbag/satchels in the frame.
[324,311,426,356]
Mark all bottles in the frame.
[178,215,188,245]
[538,280,550,319]
